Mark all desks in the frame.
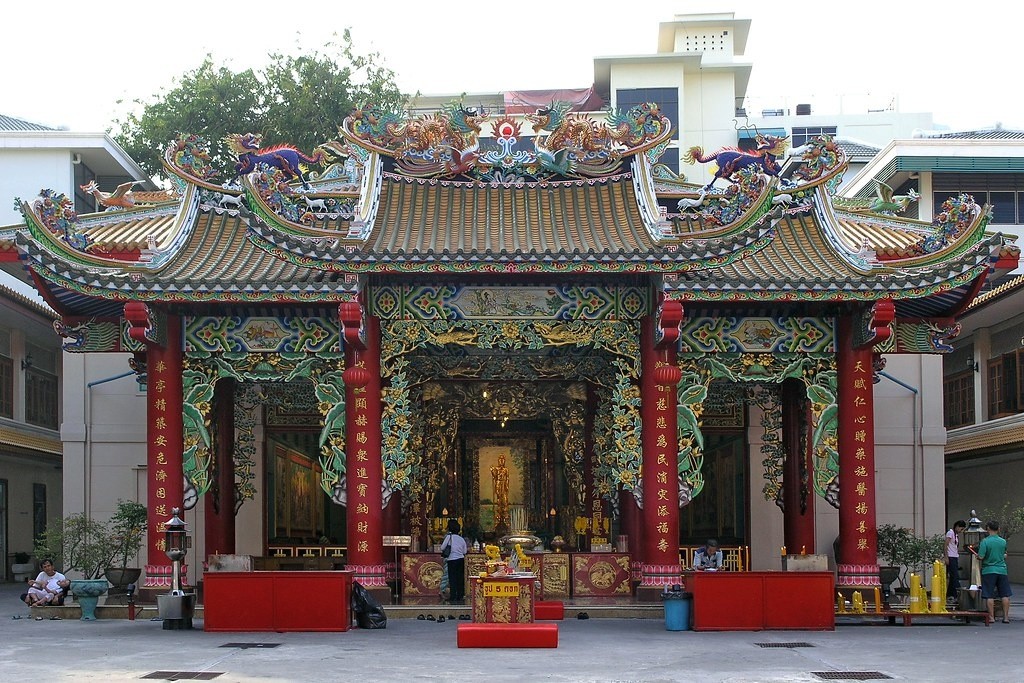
[836,612,989,627]
[681,570,835,631]
[402,552,635,598]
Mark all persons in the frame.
[944,520,967,599]
[20,559,71,607]
[440,519,467,600]
[976,521,1012,623]
[489,455,510,528]
[694,540,722,571]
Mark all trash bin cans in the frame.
[661,591,693,633]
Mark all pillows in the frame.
[746,545,750,572]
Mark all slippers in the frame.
[1002,619,1009,623]
[983,619,995,623]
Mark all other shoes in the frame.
[427,614,436,621]
[11,614,23,620]
[465,615,471,620]
[437,614,445,622]
[457,600,465,605]
[35,616,44,620]
[417,614,425,620]
[946,595,958,604]
[26,614,31,619]
[447,600,457,604]
[448,614,455,619]
[459,614,464,620]
[50,615,62,620]
[577,612,589,619]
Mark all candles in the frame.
[930,559,950,612]
[910,573,923,613]
[837,591,847,613]
[874,586,881,611]
[851,591,863,613]
[738,546,742,571]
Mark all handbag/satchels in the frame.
[441,533,452,558]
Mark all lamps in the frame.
[160,507,191,560]
[963,510,987,555]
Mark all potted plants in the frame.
[70,544,108,620]
[104,497,147,586]
[876,524,946,596]
